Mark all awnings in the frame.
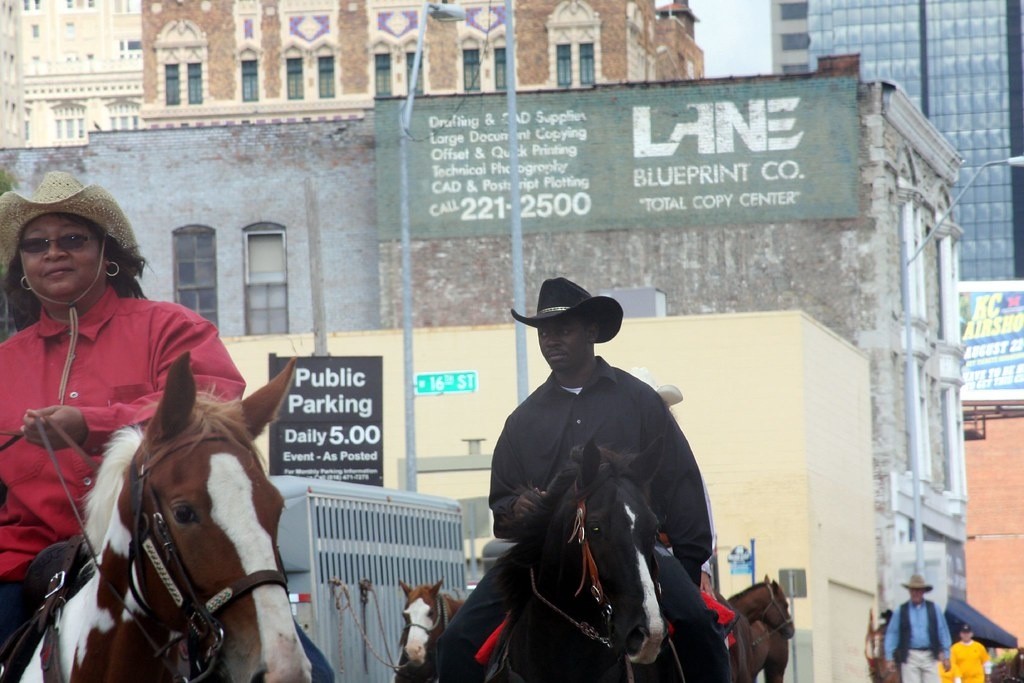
[944,595,1017,648]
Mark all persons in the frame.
[0,170,335,683]
[432,276,730,683]
[870,576,993,683]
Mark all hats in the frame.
[960,624,973,633]
[901,574,934,591]
[0,171,141,274]
[880,608,894,618]
[511,276,623,343]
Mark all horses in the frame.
[394,579,465,683]
[719,575,795,682]
[486,433,731,683]
[1,351,312,683]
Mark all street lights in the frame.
[398,4,474,494]
[898,155,1024,575]
